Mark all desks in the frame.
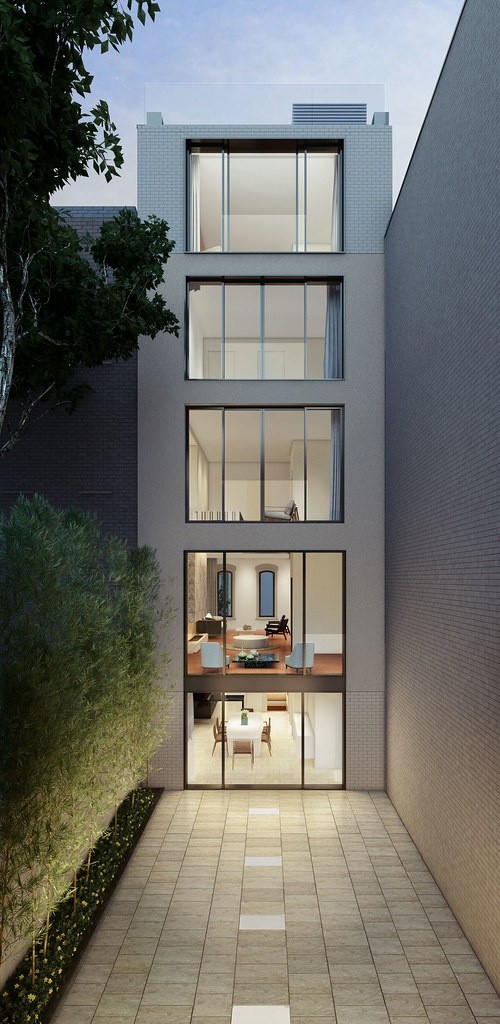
[232,654,279,668]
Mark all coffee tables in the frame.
[235,628,255,636]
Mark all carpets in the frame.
[220,644,280,651]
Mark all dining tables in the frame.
[227,714,263,757]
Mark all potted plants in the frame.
[241,710,248,725]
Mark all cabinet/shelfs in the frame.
[293,712,314,759]
[196,618,223,636]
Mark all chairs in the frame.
[285,643,314,672]
[261,718,272,756]
[265,615,290,640]
[212,718,228,756]
[232,739,254,770]
[200,642,230,671]
